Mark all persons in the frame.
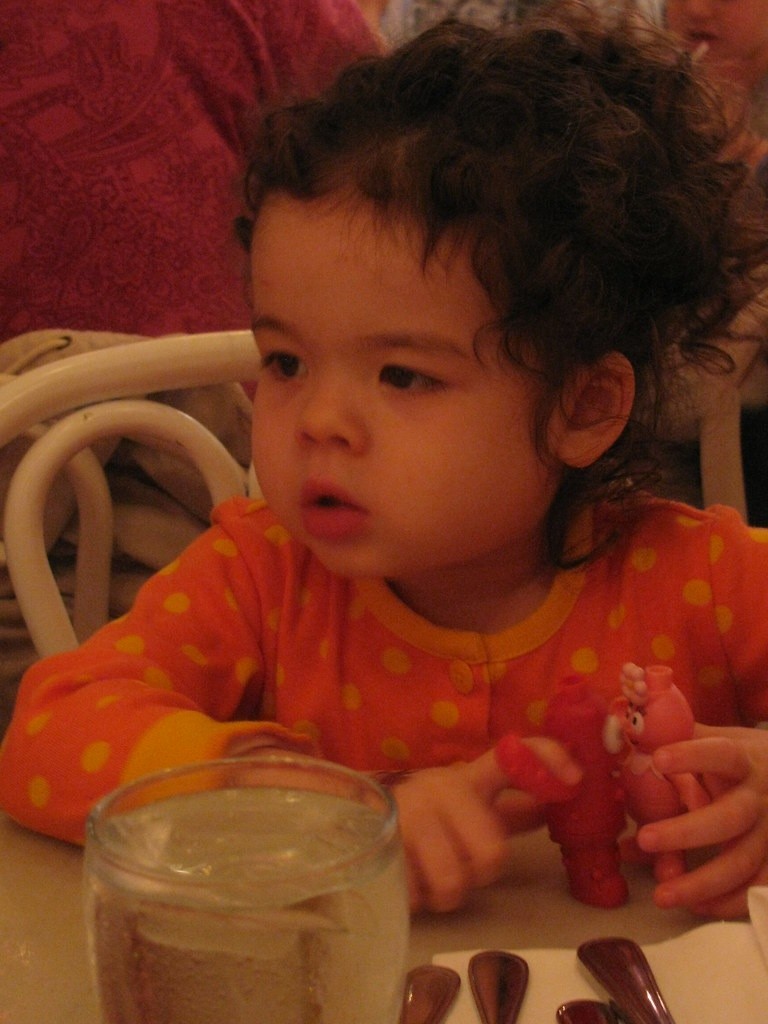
[0,22,768,922]
[0,0,768,401]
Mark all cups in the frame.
[81,755,410,1024]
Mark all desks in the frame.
[0,813,768,1024]
